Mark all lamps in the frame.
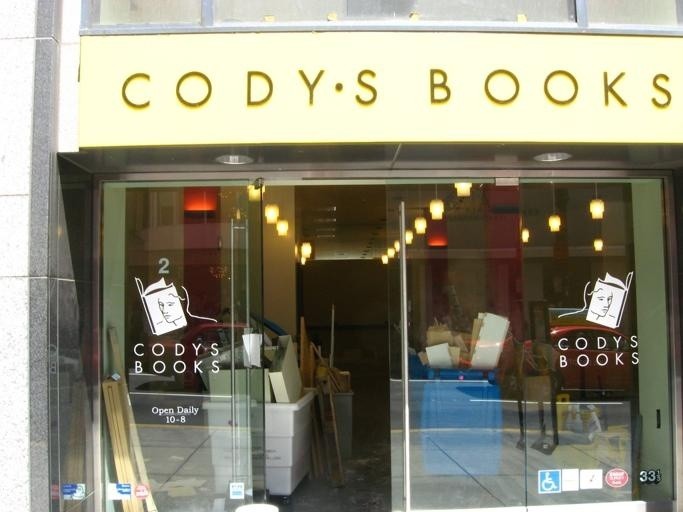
[405,183,472,245]
[520,183,605,252]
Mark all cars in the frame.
[467,322,635,406]
[135,320,252,392]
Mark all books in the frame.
[585,271,632,330]
[134,275,188,336]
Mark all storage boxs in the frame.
[201,387,317,504]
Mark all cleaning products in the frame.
[564,403,602,433]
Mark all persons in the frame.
[589,289,617,321]
[199,307,232,393]
[155,294,180,327]
